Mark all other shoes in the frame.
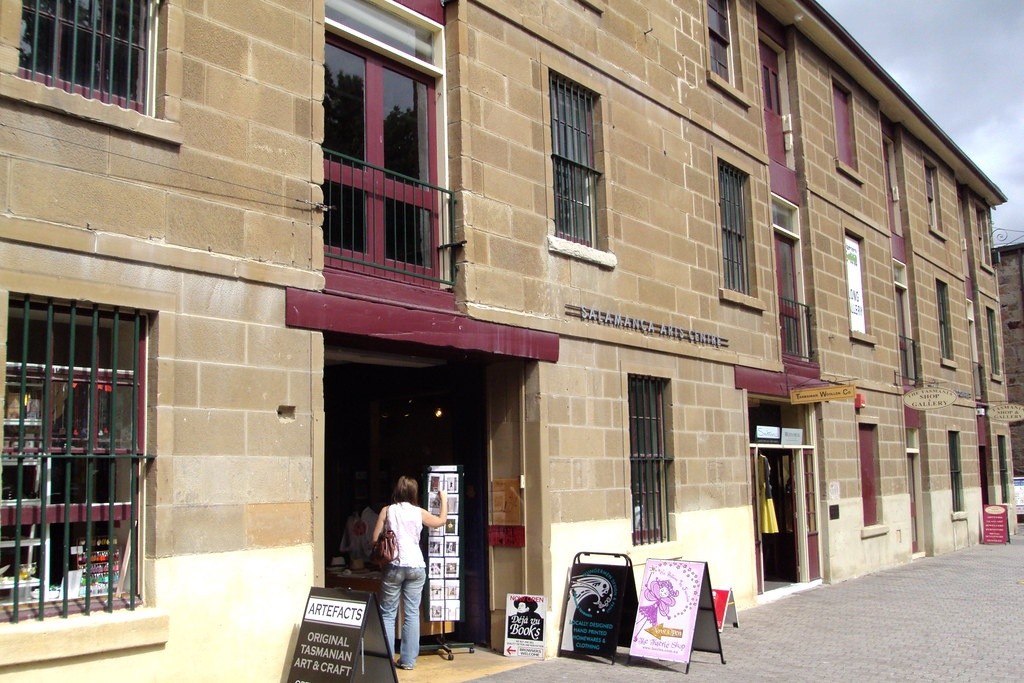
[396,659,413,670]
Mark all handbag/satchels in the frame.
[373,506,398,564]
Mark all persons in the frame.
[373,476,448,670]
